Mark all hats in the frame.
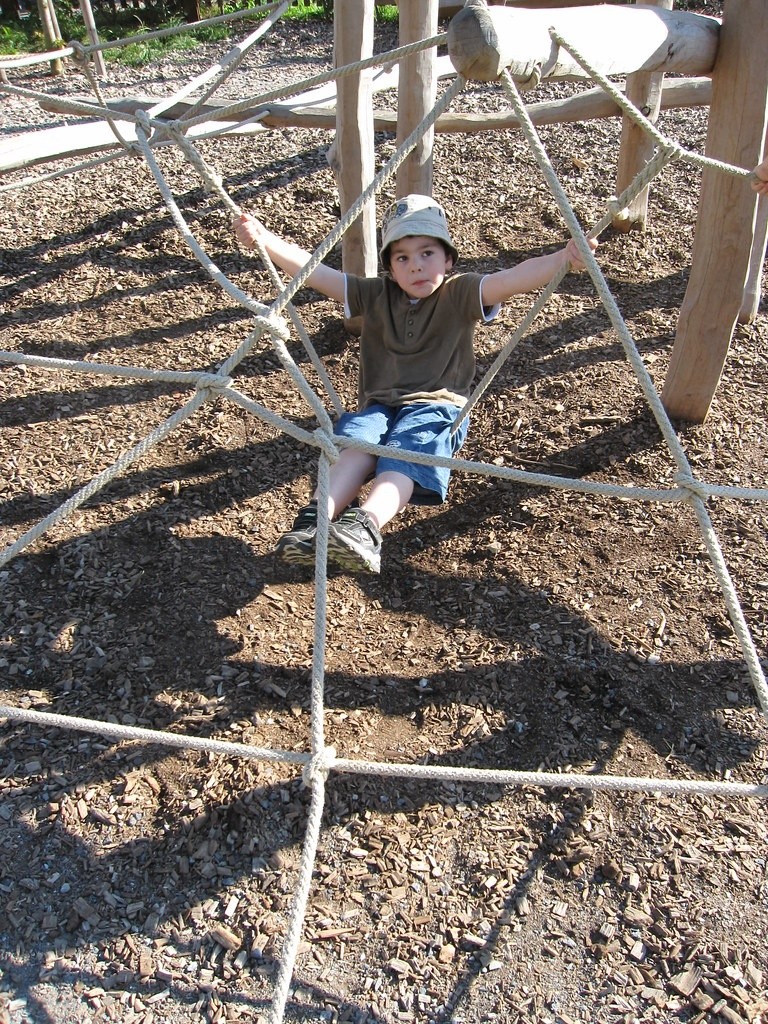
[378,194,458,271]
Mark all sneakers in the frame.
[274,499,336,566]
[312,508,383,575]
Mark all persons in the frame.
[232,194,598,574]
